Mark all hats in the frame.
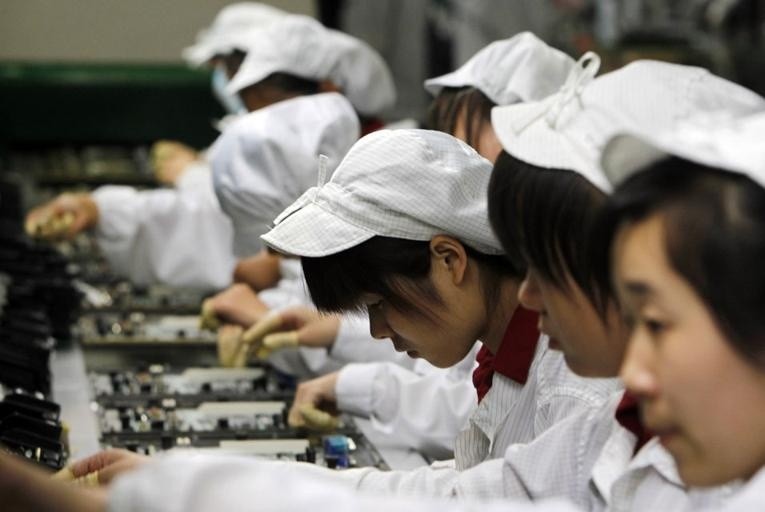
[600,65,765,191]
[223,13,398,116]
[212,91,360,257]
[200,2,290,62]
[259,128,505,259]
[423,30,594,106]
[491,49,672,193]
